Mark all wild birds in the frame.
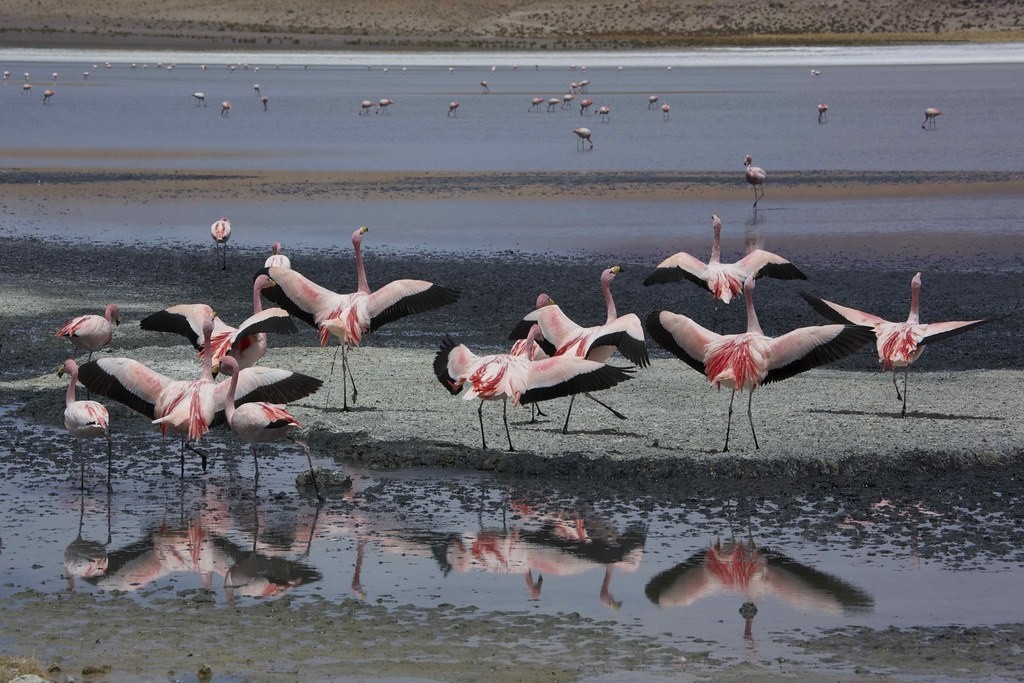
[192,84,269,114]
[76,274,324,502]
[57,358,110,492]
[433,265,653,452]
[743,154,766,209]
[817,104,828,123]
[56,303,121,363]
[367,65,407,73]
[254,226,464,410]
[800,272,1010,418]
[82,61,308,78]
[3,71,58,105]
[210,218,232,271]
[572,127,594,150]
[358,100,395,115]
[642,212,810,335]
[645,272,878,451]
[447,65,673,120]
[811,70,822,76]
[63,490,876,650]
[921,109,941,129]
